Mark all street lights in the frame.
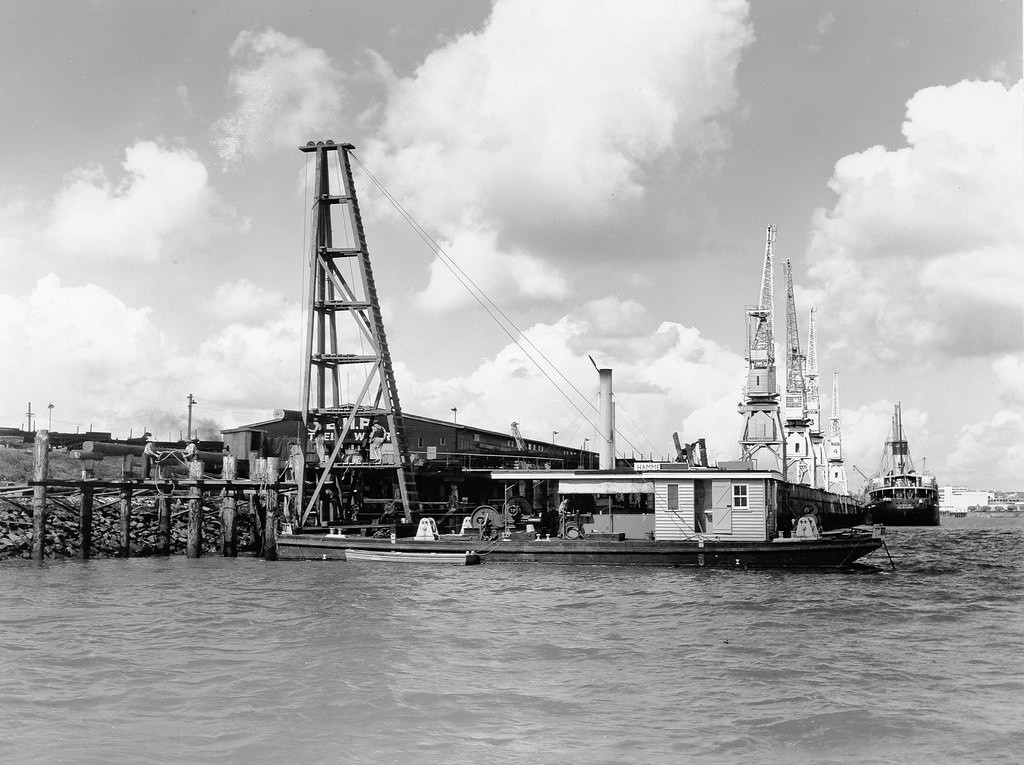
[187,400,197,440]
[48,402,54,431]
[552,431,559,444]
[449,406,457,424]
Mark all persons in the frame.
[369,420,387,464]
[184,438,197,461]
[287,442,301,481]
[140,437,161,481]
[308,418,326,464]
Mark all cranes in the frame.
[737,222,848,496]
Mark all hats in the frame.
[184,438,193,443]
[146,437,154,442]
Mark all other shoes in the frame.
[144,476,150,480]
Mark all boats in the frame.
[344,549,481,566]
[853,402,940,525]
[273,141,887,566]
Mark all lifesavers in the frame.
[384,502,396,515]
[562,520,585,541]
